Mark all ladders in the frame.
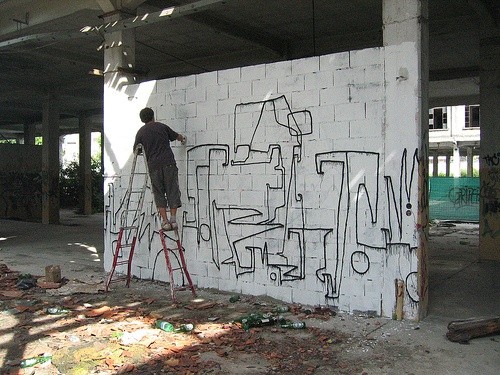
[104,143,197,303]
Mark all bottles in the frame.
[47,308,71,314]
[230,295,240,302]
[175,323,195,332]
[157,320,174,332]
[241,306,305,331]
[20,356,51,367]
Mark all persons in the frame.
[132,107,183,231]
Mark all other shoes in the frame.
[161,220,178,231]
